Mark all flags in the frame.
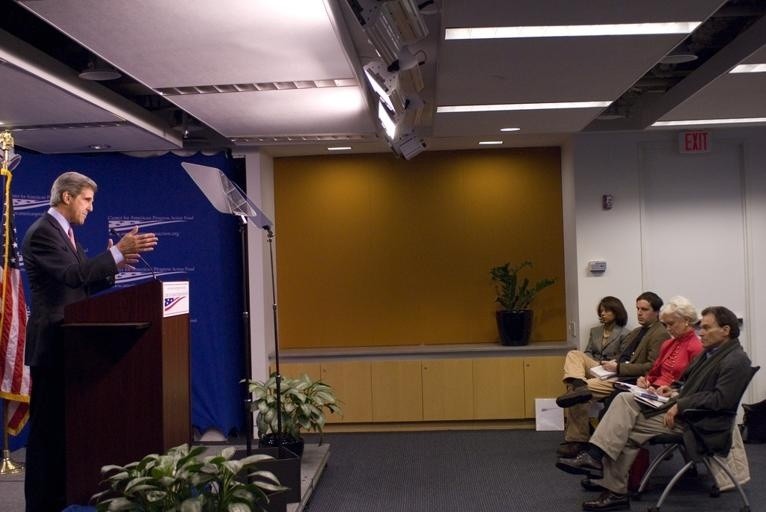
[0,169,30,437]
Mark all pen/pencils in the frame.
[611,359,616,363]
[645,373,648,393]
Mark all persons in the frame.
[555,305,750,511]
[20,171,161,511]
[554,290,705,492]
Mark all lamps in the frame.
[362,0,432,162]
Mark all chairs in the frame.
[632,365,760,512]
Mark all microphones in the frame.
[111,228,154,275]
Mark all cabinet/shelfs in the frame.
[280,357,372,430]
[474,357,568,430]
[372,357,474,430]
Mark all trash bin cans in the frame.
[630,450,649,493]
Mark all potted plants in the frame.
[488,260,555,347]
[239,371,345,459]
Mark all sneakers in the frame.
[558,440,586,456]
[555,452,601,479]
[576,474,630,512]
[555,382,593,409]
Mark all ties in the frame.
[672,346,720,397]
[617,324,651,370]
[67,227,79,253]
